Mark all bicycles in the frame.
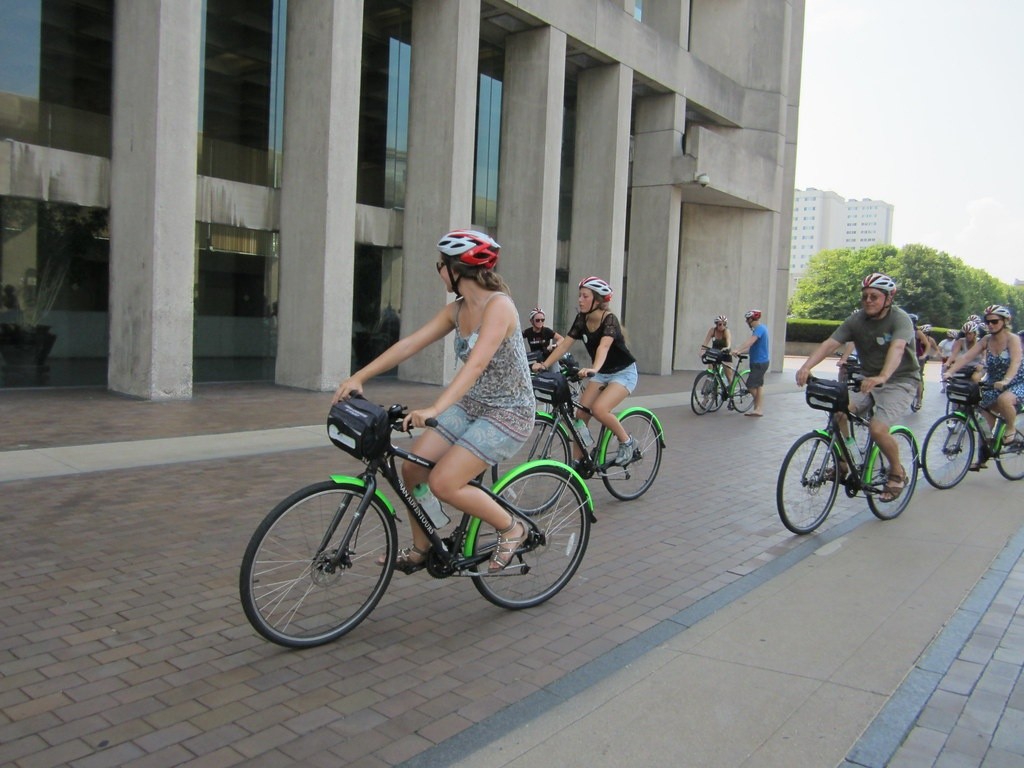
[777,352,923,534]
[922,366,1024,490]
[691,345,756,415]
[491,344,665,516]
[239,385,597,648]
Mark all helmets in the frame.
[437,229,501,272]
[579,276,614,303]
[907,305,1011,339]
[862,273,897,297]
[745,310,761,319]
[714,315,728,323]
[530,308,545,320]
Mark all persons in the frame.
[532,276,638,466]
[523,308,564,372]
[796,273,920,503]
[837,305,1024,467]
[699,315,733,408]
[732,310,770,415]
[332,229,536,572]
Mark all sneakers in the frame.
[571,460,578,467]
[615,432,638,466]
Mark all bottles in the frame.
[722,374,729,387]
[846,435,862,465]
[413,483,450,530]
[575,419,594,447]
[978,417,992,439]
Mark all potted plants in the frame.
[355,303,389,366]
[1,252,69,369]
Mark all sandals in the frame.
[486,516,530,572]
[879,465,908,502]
[377,544,431,567]
[813,464,846,478]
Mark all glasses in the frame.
[437,262,445,273]
[536,318,545,322]
[862,293,882,300]
[985,319,1001,324]
[718,323,726,326]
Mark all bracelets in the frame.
[541,363,547,369]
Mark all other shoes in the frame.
[744,411,763,417]
[702,399,709,406]
[1002,432,1017,446]
